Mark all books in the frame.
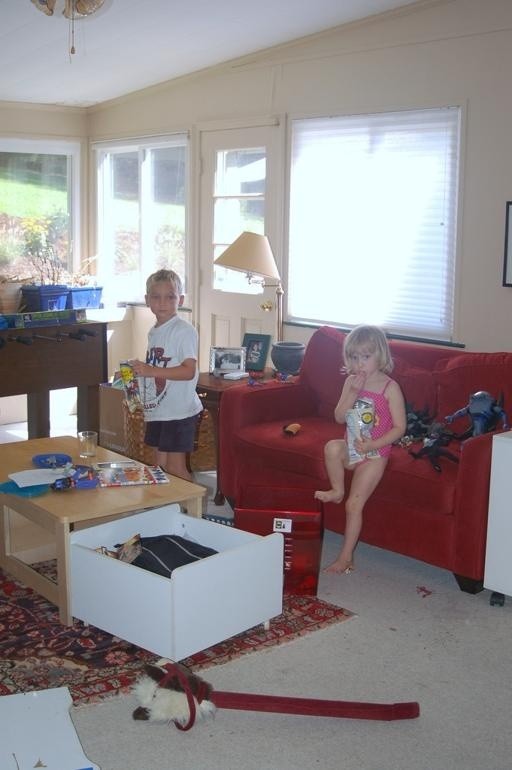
[65,504,284,663]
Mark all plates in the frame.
[0,319,109,445]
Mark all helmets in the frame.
[31,453,72,468]
[1,480,49,497]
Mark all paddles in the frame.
[0,555,356,707]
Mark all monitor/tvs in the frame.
[95,464,169,488]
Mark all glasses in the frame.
[213,232,280,282]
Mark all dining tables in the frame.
[65,252,107,310]
[19,209,69,312]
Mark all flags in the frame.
[0,283,26,313]
[271,341,305,373]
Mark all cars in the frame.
[77,431,99,457]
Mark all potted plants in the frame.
[210,346,246,374]
[501,200,512,286]
[240,333,271,371]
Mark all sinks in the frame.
[217,326,512,595]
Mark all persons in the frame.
[314,325,406,573]
[132,269,204,483]
